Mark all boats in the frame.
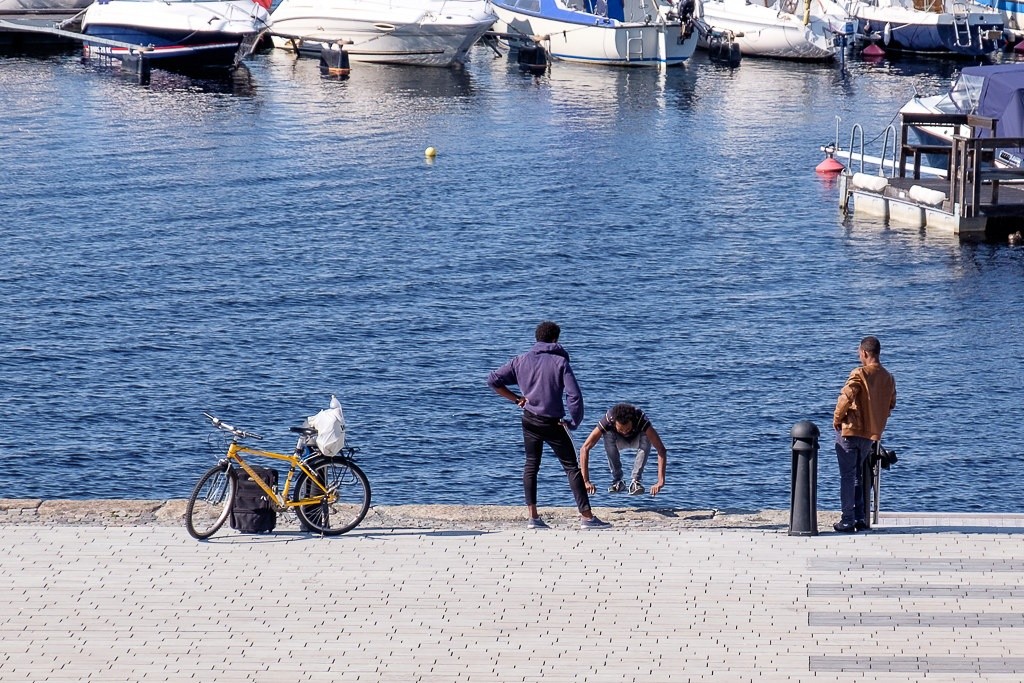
[483,0,741,69]
[672,0,854,63]
[843,0,1010,59]
[0,0,89,53]
[938,0,1024,47]
[263,0,501,69]
[896,60,1023,181]
[76,0,274,83]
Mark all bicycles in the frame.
[182,409,373,536]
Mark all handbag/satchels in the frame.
[308,409,345,457]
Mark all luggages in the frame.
[230,465,278,534]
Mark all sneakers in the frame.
[581,516,612,529]
[527,517,551,529]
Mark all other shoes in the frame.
[608,480,626,492]
[854,519,867,530]
[628,481,645,495]
[833,521,854,532]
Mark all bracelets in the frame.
[515,396,521,404]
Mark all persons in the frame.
[833,336,897,532]
[487,322,613,529]
[579,404,667,496]
